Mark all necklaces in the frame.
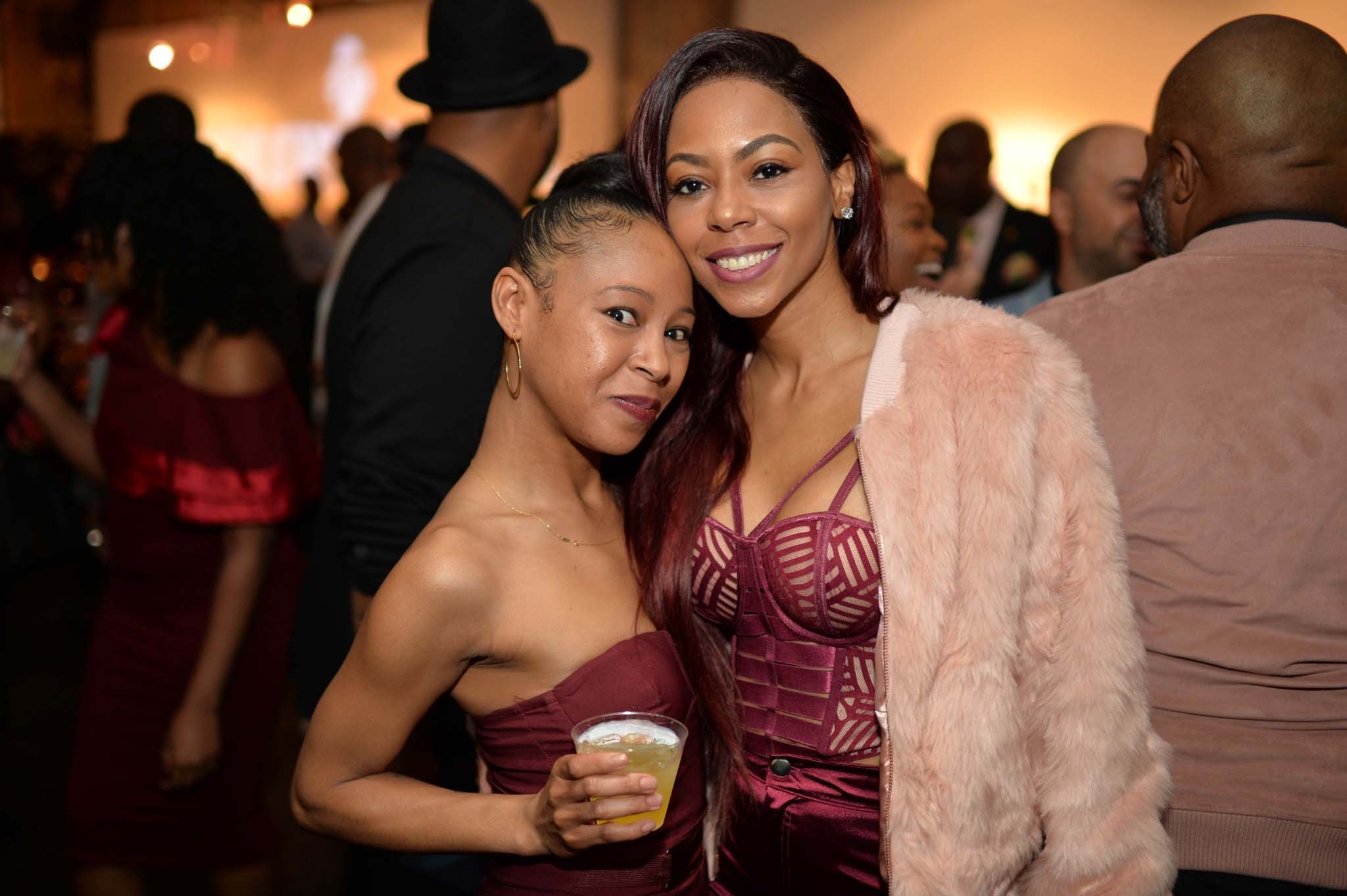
[470,459,625,545]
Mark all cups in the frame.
[570,712,689,831]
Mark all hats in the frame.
[398,0,589,110]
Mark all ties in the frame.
[941,227,960,273]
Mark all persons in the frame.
[987,126,1153,318]
[0,0,1347,896]
